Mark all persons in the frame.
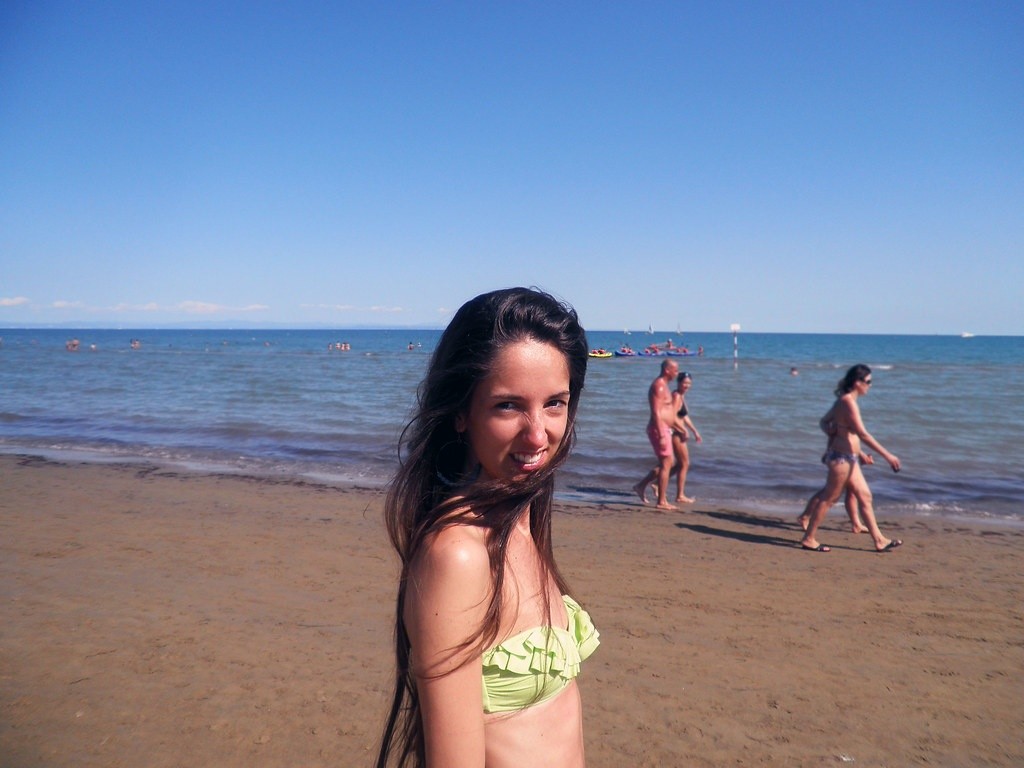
[797,364,904,553]
[633,360,678,510]
[592,348,606,354]
[650,371,701,503]
[129,338,140,349]
[791,367,798,374]
[620,346,631,353]
[65,338,79,350]
[328,341,350,350]
[644,339,688,354]
[698,345,703,355]
[377,286,600,768]
[408,341,421,350]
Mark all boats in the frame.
[589,350,613,358]
[615,350,638,356]
[668,350,697,357]
[639,351,666,357]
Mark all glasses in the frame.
[863,379,872,384]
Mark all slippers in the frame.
[804,544,831,552]
[879,538,903,550]
